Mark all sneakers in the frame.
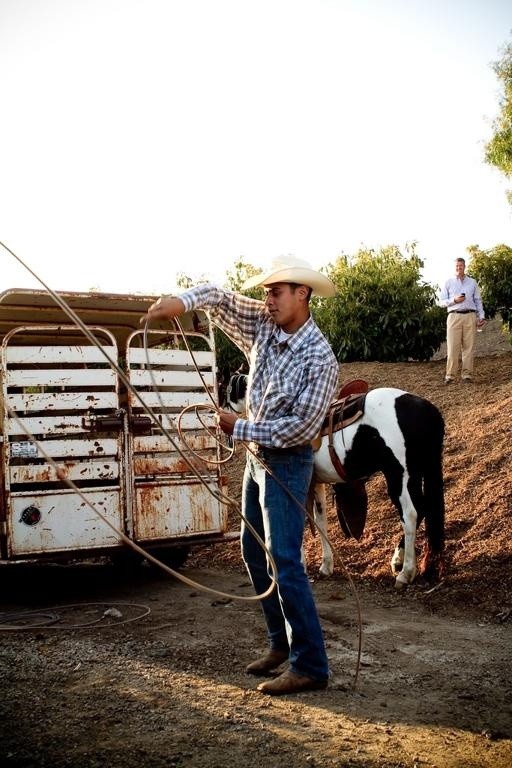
[443,377,475,385]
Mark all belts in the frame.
[448,309,477,314]
[248,442,313,453]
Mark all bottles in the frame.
[476,319,482,332]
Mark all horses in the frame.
[216,358,446,590]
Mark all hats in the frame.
[238,252,338,299]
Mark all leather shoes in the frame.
[246,644,291,674]
[255,665,329,695]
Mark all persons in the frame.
[139,253,344,697]
[434,256,486,387]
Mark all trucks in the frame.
[0,288,229,574]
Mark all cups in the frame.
[461,292,465,298]
